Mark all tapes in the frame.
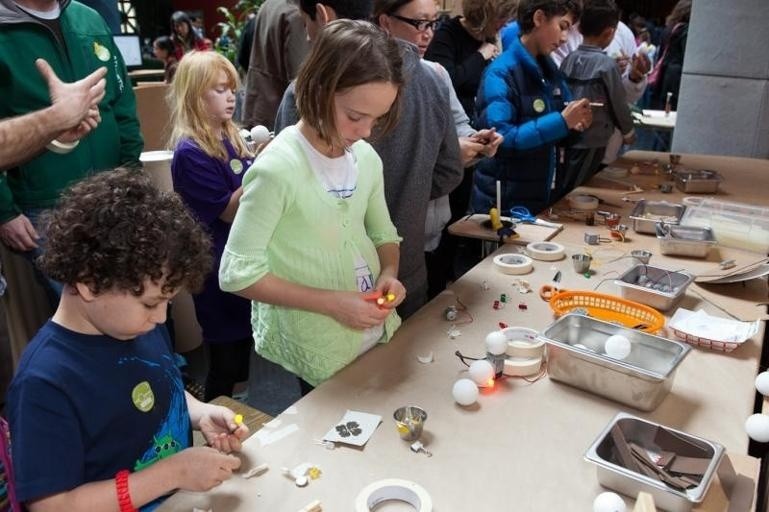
[604,167,629,178]
[500,326,545,359]
[526,241,565,261]
[502,340,543,377]
[493,253,533,275]
[44,139,81,154]
[355,478,433,512]
[568,195,599,210]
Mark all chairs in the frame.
[0,235,274,457]
[132,83,178,154]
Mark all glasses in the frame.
[387,13,441,32]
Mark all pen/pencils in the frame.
[563,101,604,107]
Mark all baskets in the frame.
[548,290,666,341]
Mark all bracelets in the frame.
[113,468,142,512]
[44,135,83,159]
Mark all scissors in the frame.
[586,194,623,209]
[540,270,573,301]
[510,206,559,228]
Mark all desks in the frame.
[633,110,676,149]
[129,69,165,85]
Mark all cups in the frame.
[393,405,427,441]
[607,225,628,241]
[669,152,682,164]
[658,183,672,194]
[631,250,652,265]
[605,213,622,228]
[571,254,593,273]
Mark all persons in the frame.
[5,167,247,512]
[152,0,692,405]
[0,55,109,168]
[0,1,142,406]
